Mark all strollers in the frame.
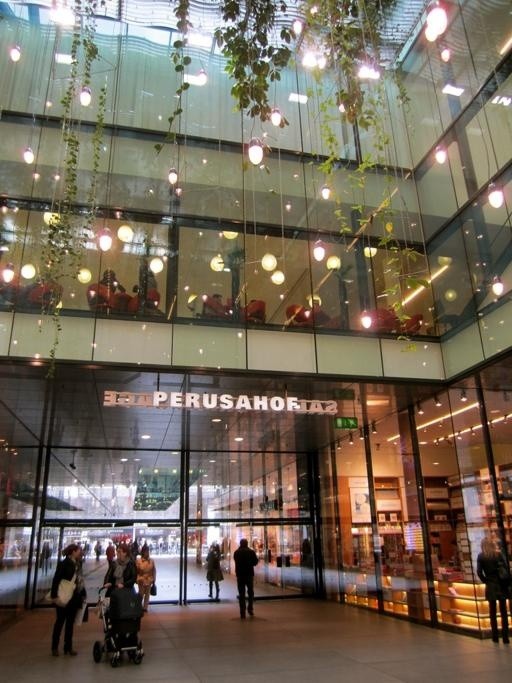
[93,584,145,667]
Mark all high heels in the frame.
[65,650,76,655]
[52,649,58,656]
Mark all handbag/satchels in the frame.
[45,579,76,608]
[497,562,511,579]
[151,586,156,595]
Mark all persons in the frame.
[0,262,222,316]
[50,535,258,656]
[477,536,511,643]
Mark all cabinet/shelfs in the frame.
[338,463,511,630]
